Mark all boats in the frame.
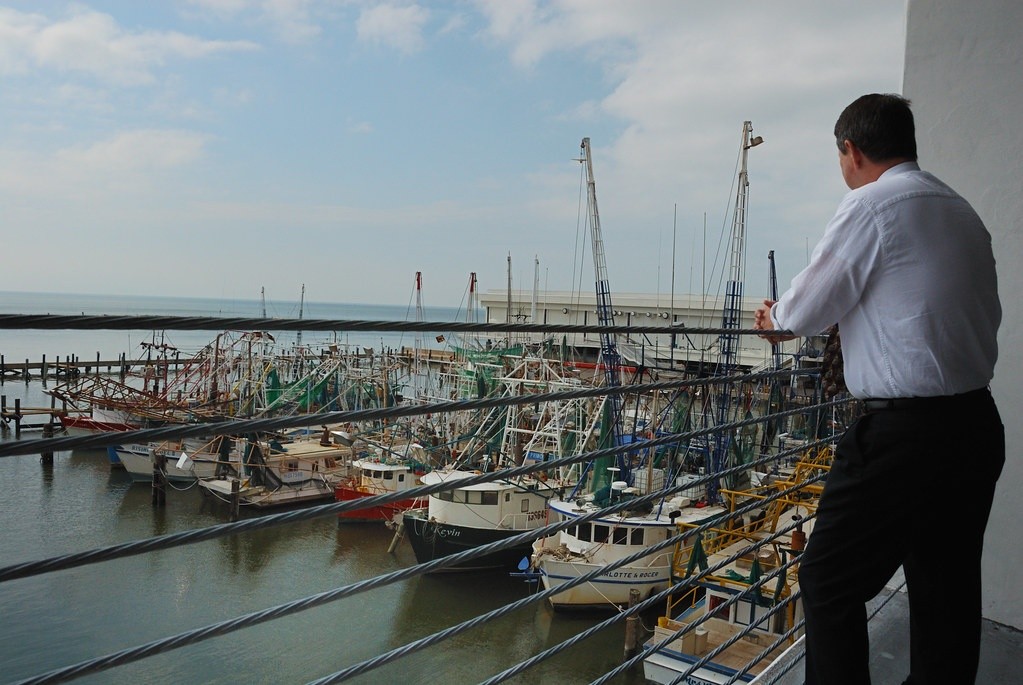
[45,203,851,685]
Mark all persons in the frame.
[758,93,1005,685]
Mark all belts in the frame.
[864,391,990,410]
